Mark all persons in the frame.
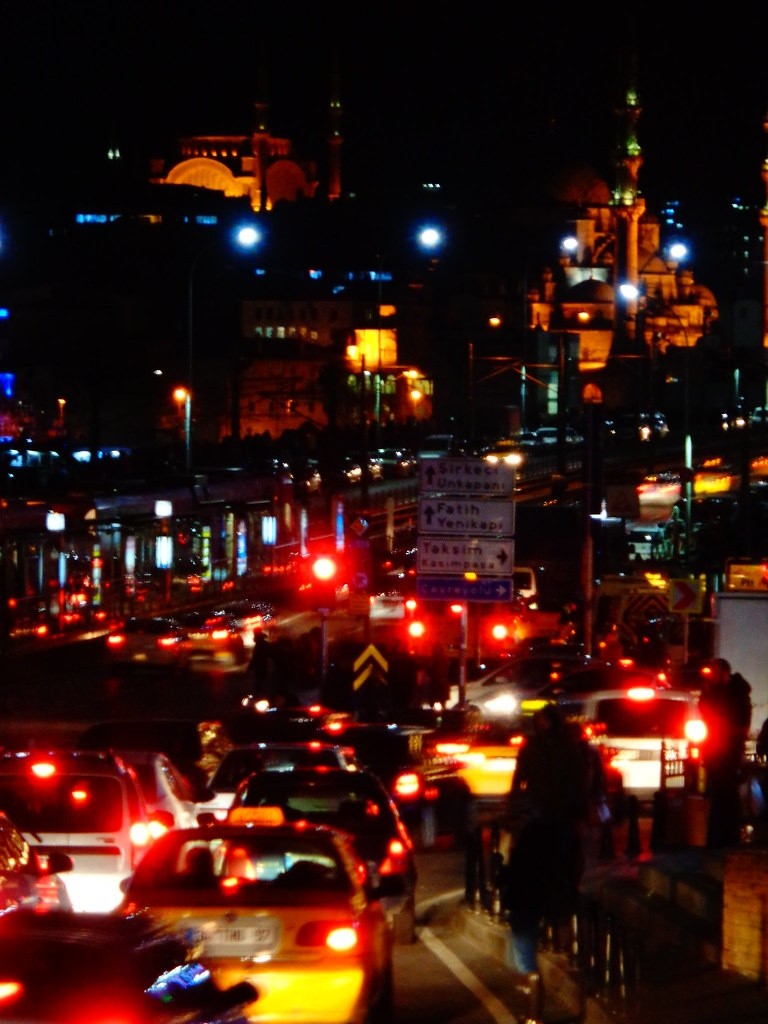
[507,705,601,971]
[697,658,754,856]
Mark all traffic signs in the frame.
[415,459,516,601]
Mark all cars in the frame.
[0,901,263,1024]
[1,746,195,918]
[635,470,684,504]
[118,821,395,1024]
[692,458,746,498]
[103,599,278,677]
[429,646,704,804]
[263,424,571,503]
[194,696,464,943]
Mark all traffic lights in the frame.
[406,594,429,646]
[489,613,512,644]
[298,532,354,610]
[175,388,192,472]
